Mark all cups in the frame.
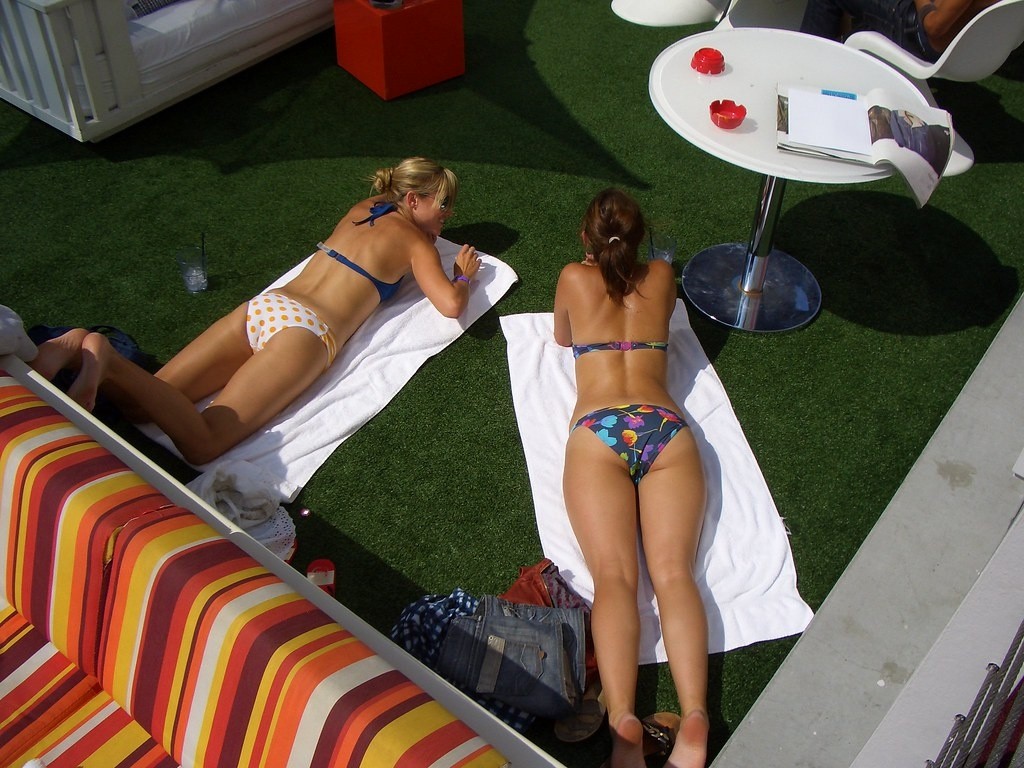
[646,233,677,266]
[176,246,207,293]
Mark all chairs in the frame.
[844,0,1024,176]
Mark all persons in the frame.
[553,189,709,768]
[800,0,1000,62]
[30,156,481,465]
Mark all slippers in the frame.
[600,713,680,768]
[553,680,606,744]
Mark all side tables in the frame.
[333,0,467,100]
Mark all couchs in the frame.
[0,1,334,143]
[1,376,513,768]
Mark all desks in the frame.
[648,27,931,335]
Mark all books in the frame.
[776,85,953,209]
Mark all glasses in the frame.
[422,194,449,210]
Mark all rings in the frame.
[469,249,474,253]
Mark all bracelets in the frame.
[452,275,472,289]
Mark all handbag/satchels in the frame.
[434,592,586,719]
[391,590,532,735]
[496,558,598,681]
